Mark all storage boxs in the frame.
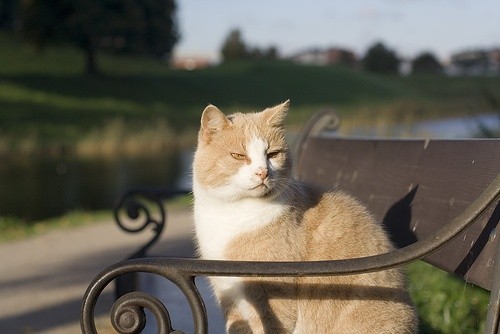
[80,108,500,334]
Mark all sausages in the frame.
[191,99,418,333]
